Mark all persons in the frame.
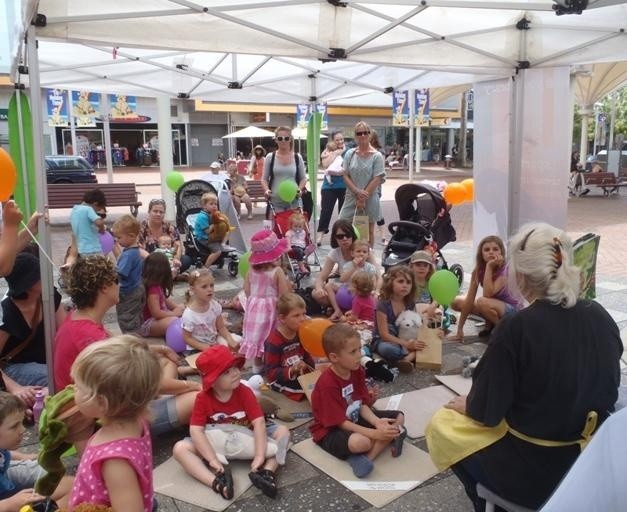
[0,188,228,331]
[591,160,602,173]
[233,219,447,371]
[262,126,307,228]
[316,121,385,252]
[0,254,458,512]
[424,222,623,512]
[446,236,530,344]
[569,151,590,196]
[200,145,266,220]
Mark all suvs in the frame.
[43,154,98,185]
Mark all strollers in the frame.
[379,180,464,291]
[263,187,324,289]
[170,178,241,279]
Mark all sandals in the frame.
[249,467,276,498]
[212,469,233,499]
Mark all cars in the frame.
[577,149,627,174]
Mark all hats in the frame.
[411,251,432,265]
[4,253,40,296]
[252,145,266,156]
[195,345,245,392]
[248,230,289,265]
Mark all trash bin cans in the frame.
[444,155,452,169]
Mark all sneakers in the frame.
[348,453,372,478]
[397,360,414,372]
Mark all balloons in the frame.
[166,169,184,192]
[0,149,16,202]
[444,182,467,205]
[429,269,457,307]
[461,179,474,202]
[279,179,297,203]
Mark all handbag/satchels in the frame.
[302,188,313,220]
[353,206,369,241]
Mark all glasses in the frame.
[278,137,289,141]
[335,234,352,239]
[357,131,370,136]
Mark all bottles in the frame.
[32,389,44,437]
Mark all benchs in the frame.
[45,182,143,218]
[574,171,627,199]
[244,179,272,220]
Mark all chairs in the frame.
[474,396,626,512]
